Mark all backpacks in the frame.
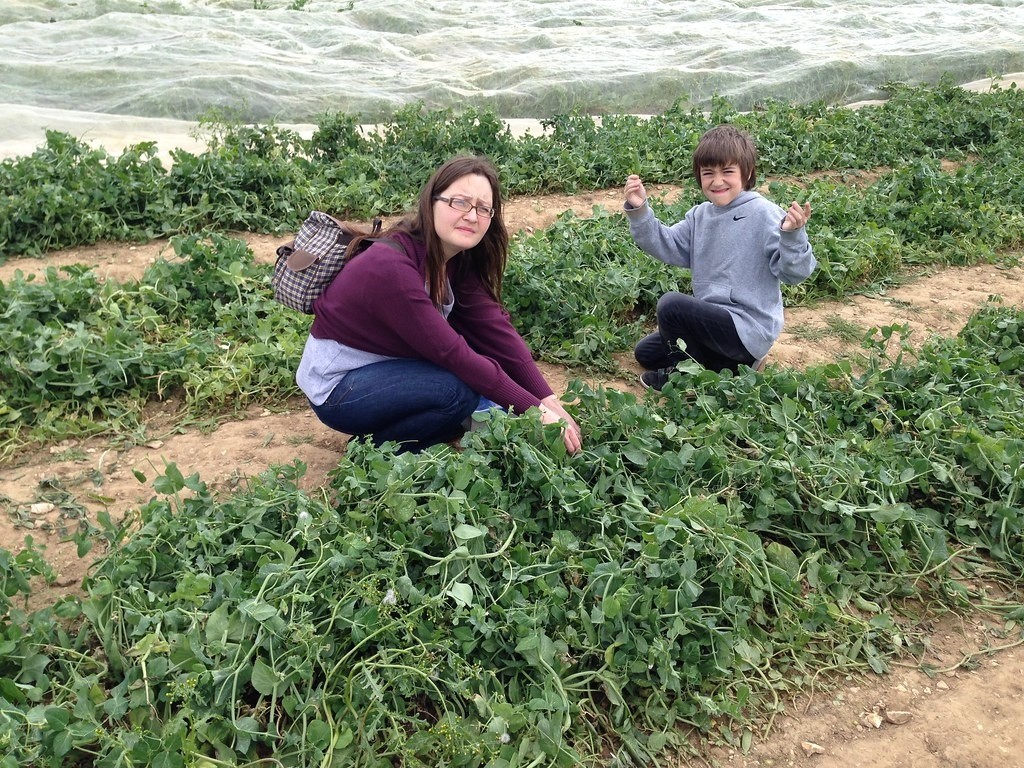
[270,210,410,314]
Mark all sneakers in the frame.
[640,368,674,397]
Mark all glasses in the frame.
[434,194,495,219]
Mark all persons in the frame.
[623,125,817,397]
[295,154,583,474]
